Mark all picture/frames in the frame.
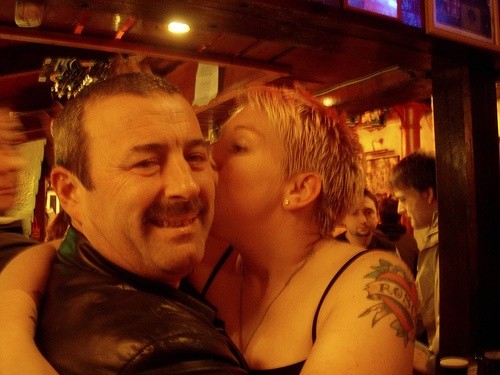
[342,0,403,23]
[426,0,500,53]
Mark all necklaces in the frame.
[237,248,312,355]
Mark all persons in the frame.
[0,86,416,375]
[377,198,419,277]
[334,188,395,252]
[0,96,40,274]
[389,152,441,359]
[31,73,248,375]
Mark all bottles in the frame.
[477,352,500,375]
[439,357,469,375]
